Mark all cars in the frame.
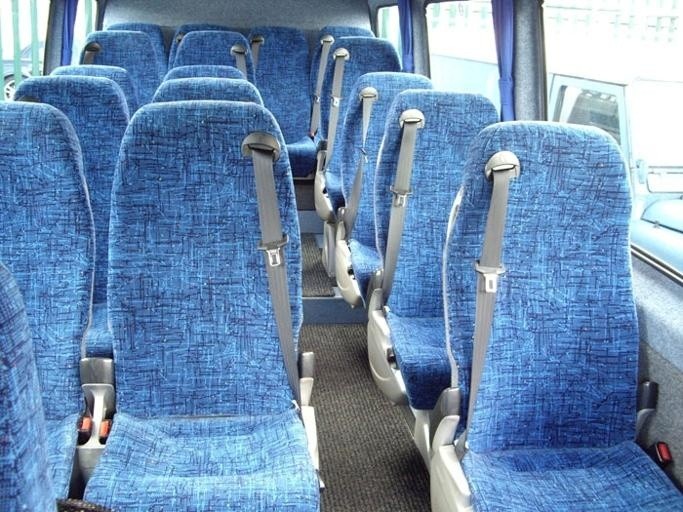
[2,39,45,99]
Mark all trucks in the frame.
[425,23,683,281]
